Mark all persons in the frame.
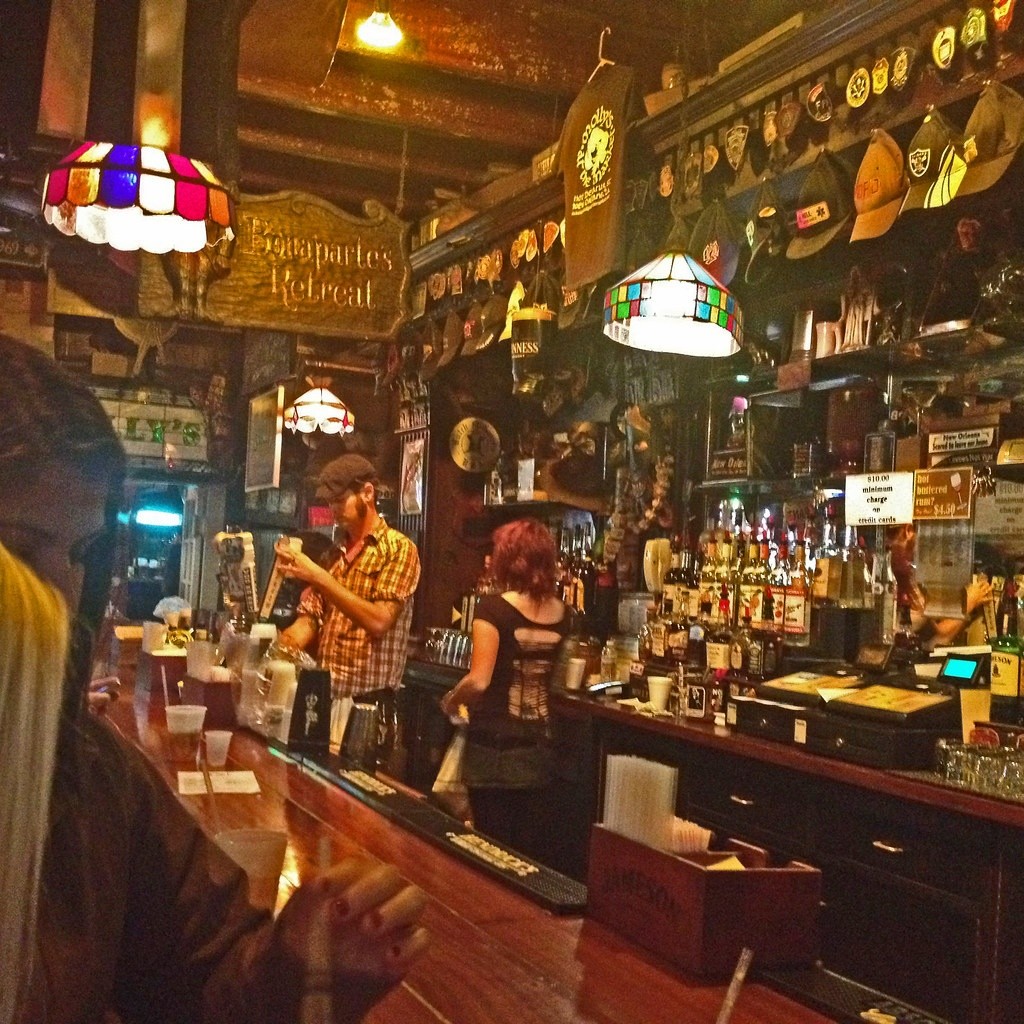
[86,674,123,717]
[0,331,431,1024]
[274,453,421,771]
[0,540,70,1024]
[440,517,571,879]
[164,525,183,596]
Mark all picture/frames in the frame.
[244,385,284,493]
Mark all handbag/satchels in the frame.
[431,716,474,827]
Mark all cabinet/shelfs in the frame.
[673,740,1024,1024]
[694,317,1024,490]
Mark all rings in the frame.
[292,558,295,562]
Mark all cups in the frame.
[648,676,673,712]
[165,704,208,763]
[215,829,289,915]
[933,738,1024,800]
[425,626,473,658]
[204,729,233,767]
[235,624,379,774]
[566,658,586,690]
[815,321,842,359]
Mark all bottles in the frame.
[989,577,1024,725]
[552,634,618,685]
[460,555,507,634]
[640,503,872,732]
[551,521,596,614]
[195,609,219,643]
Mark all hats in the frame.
[316,453,376,498]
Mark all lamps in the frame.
[283,386,356,437]
[40,141,238,256]
[603,0,746,357]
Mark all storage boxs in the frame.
[588,821,822,978]
[108,631,237,724]
